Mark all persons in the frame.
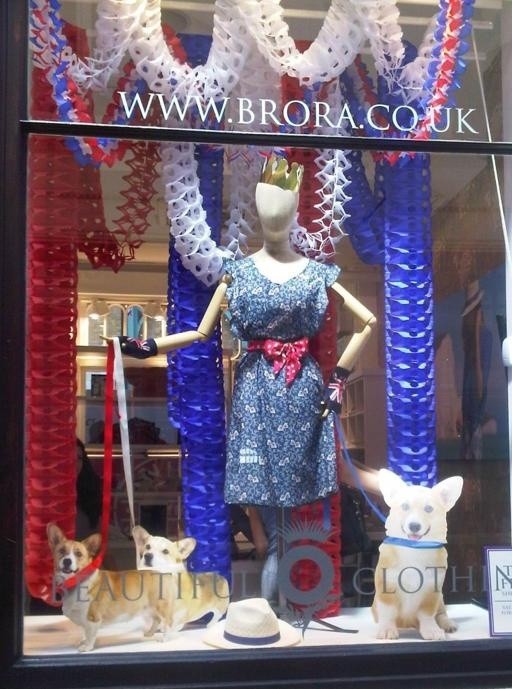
[239,504,283,608]
[77,437,100,540]
[99,184,377,619]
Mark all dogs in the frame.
[45,522,176,653]
[131,525,230,638]
[340,461,466,642]
